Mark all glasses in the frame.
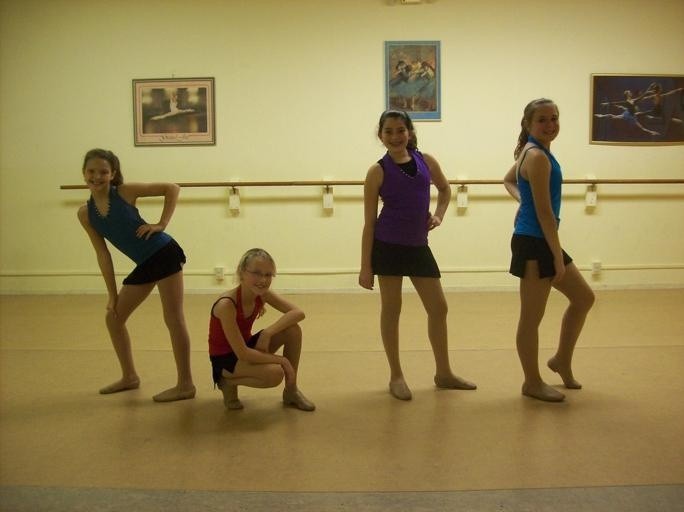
[245,268,275,278]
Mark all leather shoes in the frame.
[433,373,477,390]
[153,385,196,403]
[283,388,317,412]
[522,383,566,403]
[218,385,245,411]
[388,381,414,402]
[548,354,582,389]
[100,379,141,395]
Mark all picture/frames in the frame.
[129,76,216,150]
[587,71,684,146]
[383,38,443,124]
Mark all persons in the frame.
[208,249,316,411]
[395,60,436,111]
[612,82,684,124]
[504,99,595,401]
[594,91,663,135]
[359,110,477,399]
[77,150,195,401]
